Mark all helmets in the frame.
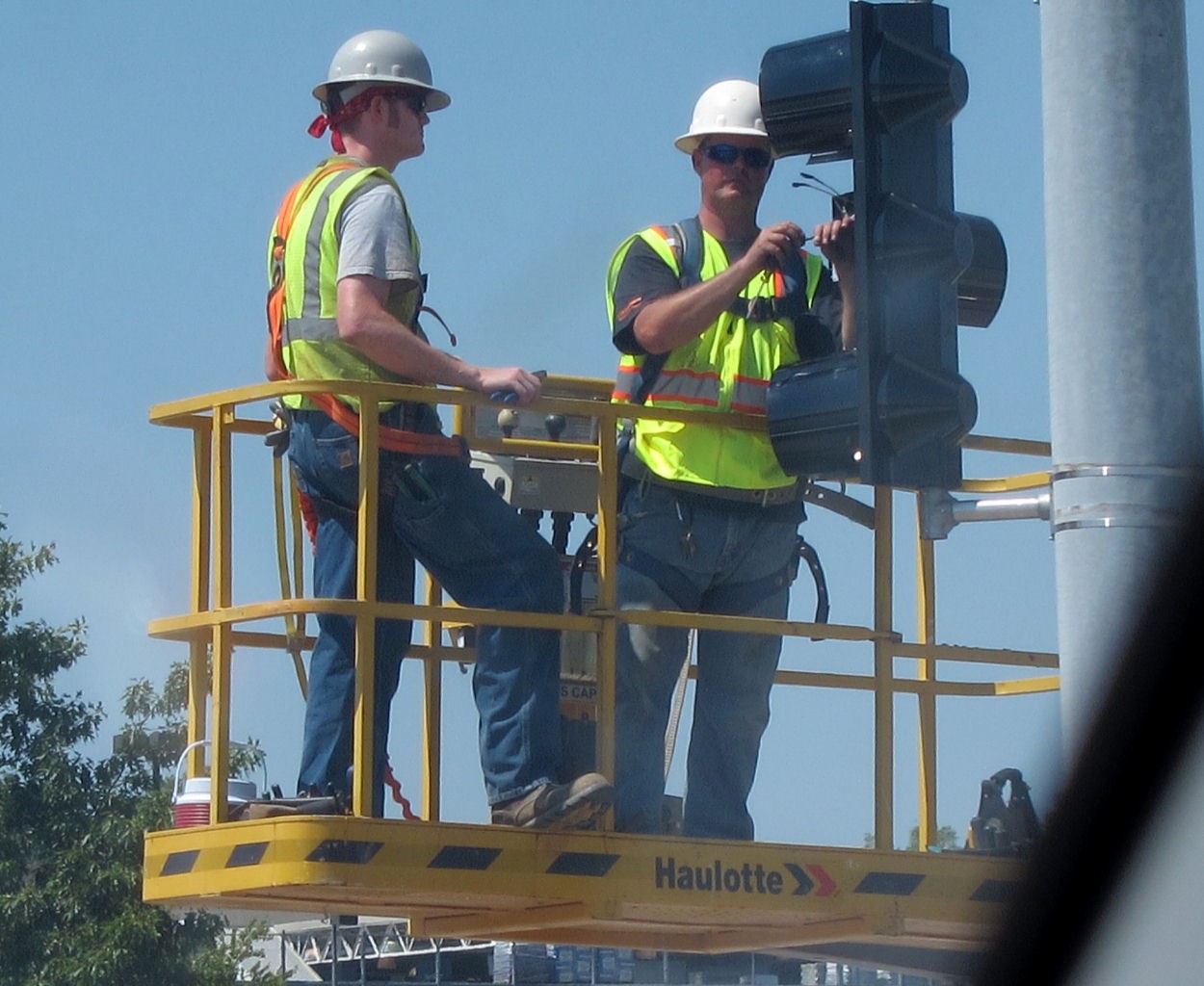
[312,29,452,113]
[672,79,771,155]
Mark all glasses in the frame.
[383,92,427,111]
[700,144,772,170]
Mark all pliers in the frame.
[489,370,547,405]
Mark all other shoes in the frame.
[490,772,617,834]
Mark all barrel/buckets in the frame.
[171,739,268,830]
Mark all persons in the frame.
[266,29,613,834]
[606,77,854,842]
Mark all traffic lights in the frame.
[758,2,1008,487]
